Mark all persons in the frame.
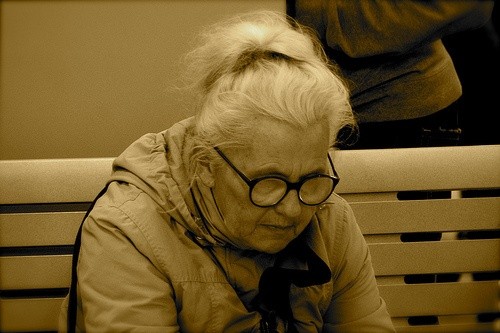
[58,10,395,333]
[286,0,500,325]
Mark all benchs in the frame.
[0,146,500,333]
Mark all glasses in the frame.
[214,144,342,208]
